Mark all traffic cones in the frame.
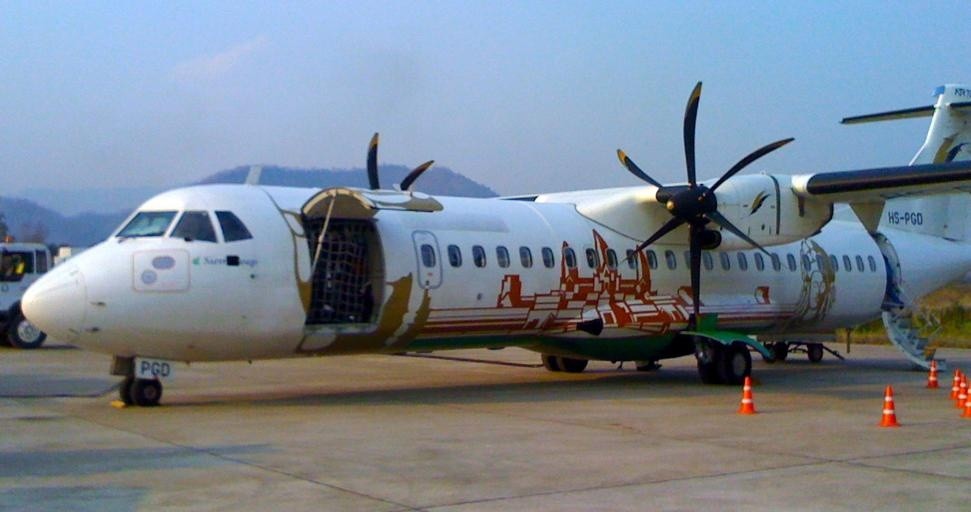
[949,367,971,418]
[881,385,901,427]
[926,359,940,387]
[738,375,759,414]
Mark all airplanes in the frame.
[9,72,970,403]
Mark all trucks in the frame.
[0,243,96,349]
